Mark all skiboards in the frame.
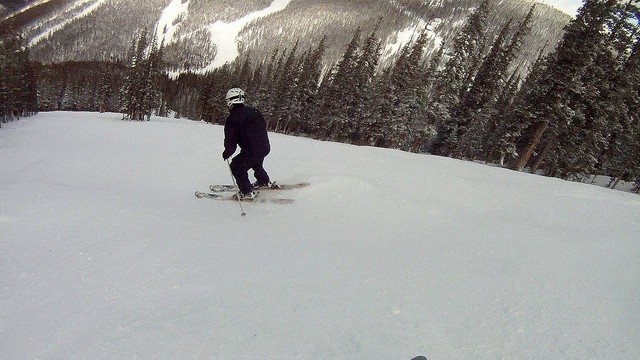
[195,182,310,204]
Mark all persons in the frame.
[221,87,271,199]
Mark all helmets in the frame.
[225,88,245,109]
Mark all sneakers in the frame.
[232,191,254,201]
[252,180,272,188]
